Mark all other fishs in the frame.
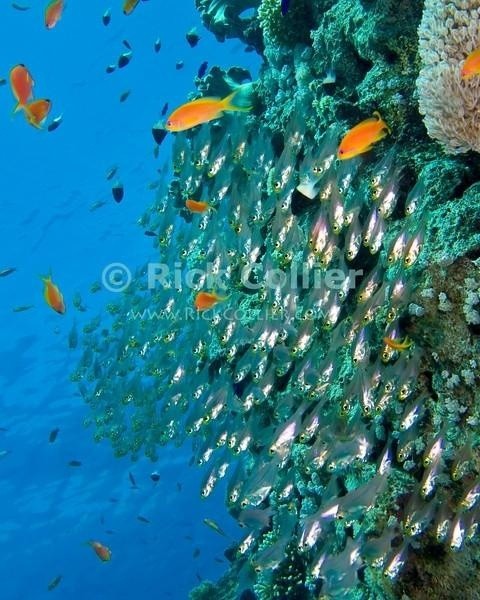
[0,0,479,600]
